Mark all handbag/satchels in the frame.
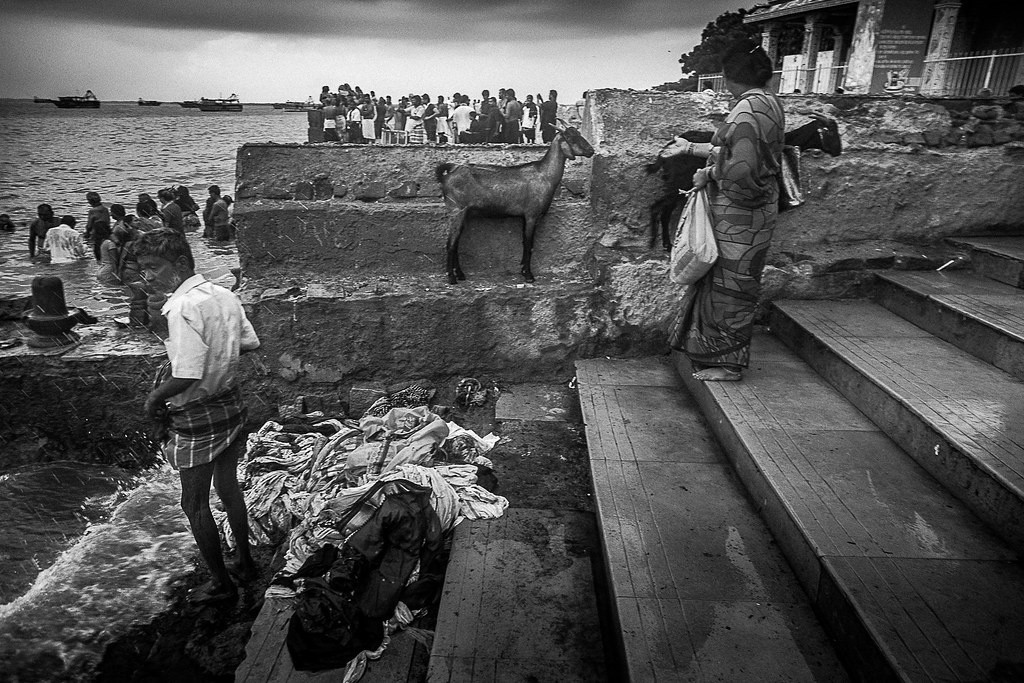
[669,185,719,286]
[768,91,806,214]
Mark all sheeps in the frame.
[641,110,843,253]
[436,114,596,286]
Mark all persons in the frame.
[977,87,993,99]
[130,226,261,604]
[702,81,715,92]
[84,184,202,286]
[792,88,802,94]
[28,203,63,258]
[202,184,237,241]
[1006,84,1024,98]
[655,38,787,382]
[0,212,17,232]
[319,82,559,144]
[42,215,88,265]
[834,87,844,94]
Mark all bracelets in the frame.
[707,164,715,182]
[687,141,694,158]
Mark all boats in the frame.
[197,93,243,112]
[51,90,101,109]
[139,98,163,106]
[177,100,199,108]
[271,95,323,112]
